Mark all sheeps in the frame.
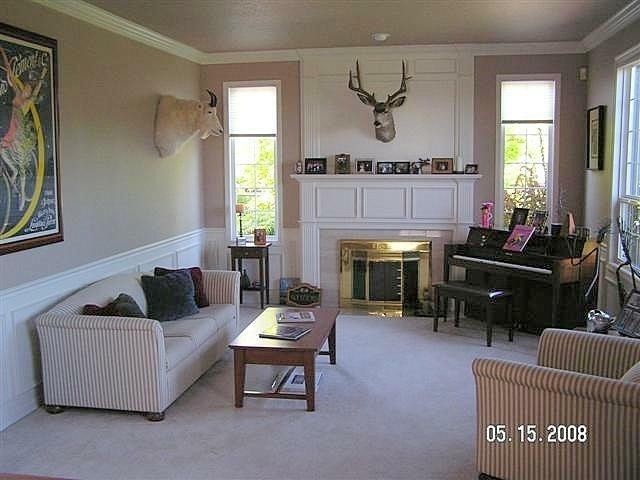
[153,88,224,158]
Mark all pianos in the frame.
[444,226,600,328]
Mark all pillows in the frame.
[154,266,209,308]
[141,270,201,320]
[84,293,145,318]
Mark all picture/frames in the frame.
[0,21,65,255]
[584,105,605,171]
[465,164,478,174]
[305,157,327,174]
[333,153,351,174]
[432,158,453,174]
[377,162,394,173]
[508,208,550,235]
[395,162,411,174]
[254,229,266,245]
[355,158,375,174]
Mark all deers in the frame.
[348,58,414,143]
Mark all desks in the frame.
[227,242,273,309]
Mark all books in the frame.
[259,323,311,341]
[275,309,316,323]
[271,370,325,395]
[500,224,536,252]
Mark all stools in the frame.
[431,281,514,345]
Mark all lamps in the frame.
[236,204,244,237]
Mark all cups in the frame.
[456,155,464,173]
[551,224,562,236]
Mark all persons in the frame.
[1,62,37,213]
[379,165,392,173]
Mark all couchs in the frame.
[471,326,639,480]
[36,269,243,422]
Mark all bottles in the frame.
[484,202,493,227]
[295,160,302,173]
